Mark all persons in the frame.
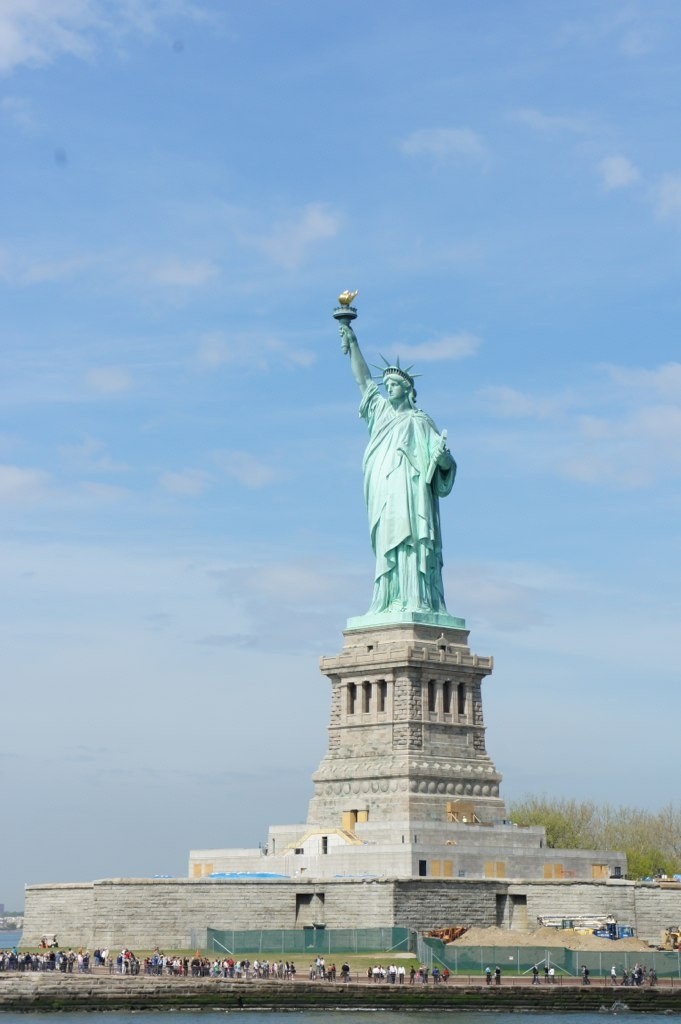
[610,966,618,986]
[338,325,457,615]
[0,938,297,981]
[494,966,501,985]
[366,964,450,985]
[309,955,352,983]
[532,964,555,985]
[581,964,590,985]
[485,966,492,985]
[621,963,658,986]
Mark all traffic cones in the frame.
[195,948,201,956]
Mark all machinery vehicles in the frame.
[535,915,633,941]
[659,925,681,950]
